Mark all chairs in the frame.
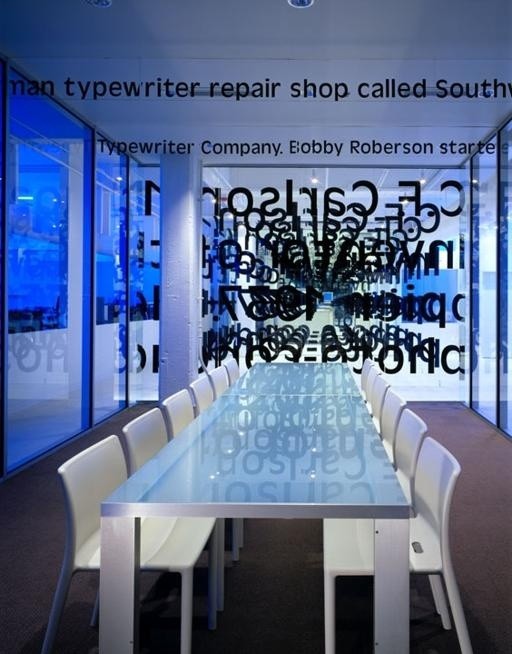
[43,359,244,654]
[323,358,473,654]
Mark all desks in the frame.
[95,361,419,653]
